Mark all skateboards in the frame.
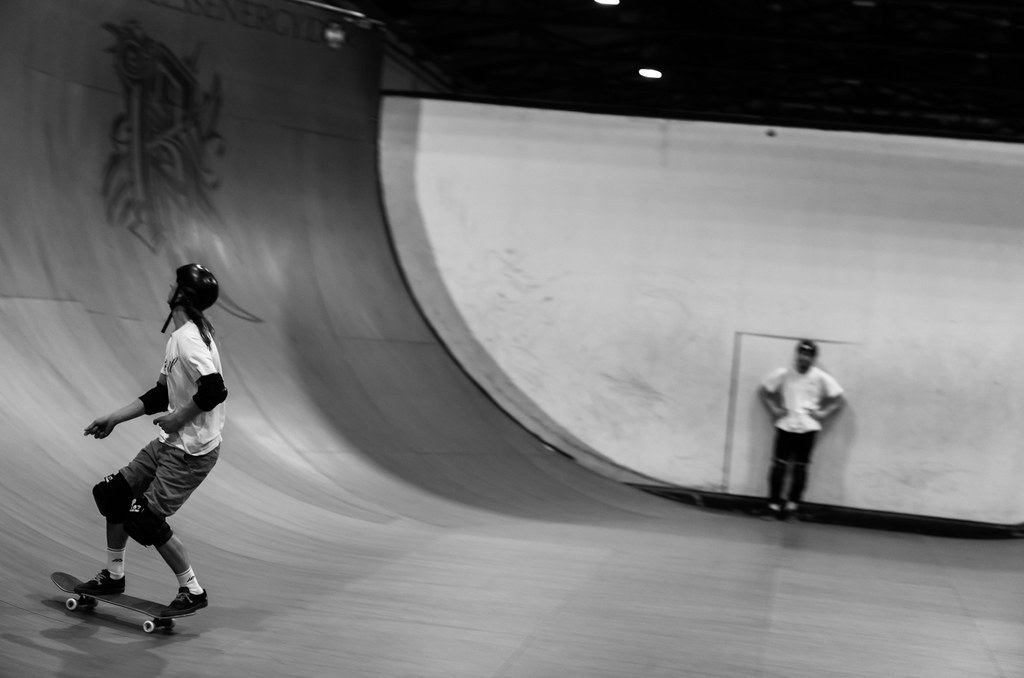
[51,569,195,634]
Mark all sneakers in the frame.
[75,569,125,595]
[161,587,208,617]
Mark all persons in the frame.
[73,263,228,617]
[758,340,844,517]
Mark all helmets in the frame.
[798,341,815,357]
[176,264,219,311]
